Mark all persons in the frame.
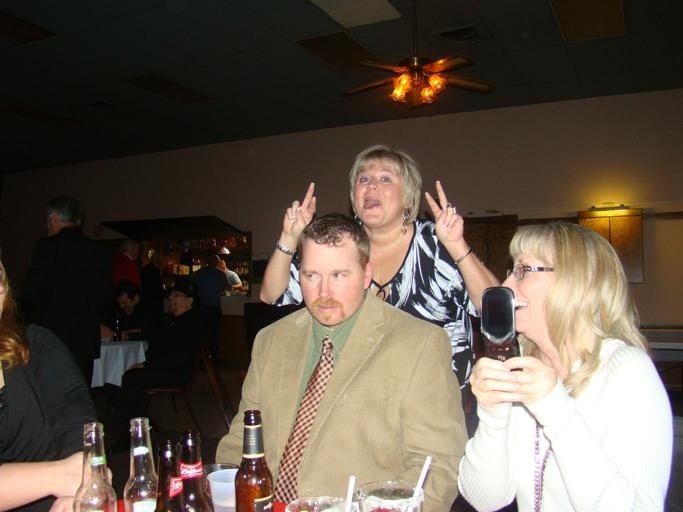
[23,198,107,383]
[0,260,114,512]
[212,214,470,512]
[456,220,675,512]
[100,238,244,419]
[260,146,502,430]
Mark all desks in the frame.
[90,341,149,390]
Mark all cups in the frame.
[285,493,354,512]
[358,479,427,511]
[202,463,238,511]
[208,467,239,511]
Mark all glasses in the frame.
[503,263,553,284]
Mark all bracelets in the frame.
[276,242,297,256]
[452,249,473,267]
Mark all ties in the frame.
[271,336,336,506]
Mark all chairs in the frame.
[200,353,238,431]
[142,348,204,431]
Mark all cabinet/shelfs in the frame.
[462,215,519,283]
[142,233,252,297]
[574,208,646,284]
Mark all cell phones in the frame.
[481,286,522,371]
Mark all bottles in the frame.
[234,408,273,511]
[112,313,121,342]
[123,418,158,512]
[152,424,212,512]
[71,419,117,512]
[240,260,248,274]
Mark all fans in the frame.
[343,1,490,97]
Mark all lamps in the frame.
[385,72,451,106]
